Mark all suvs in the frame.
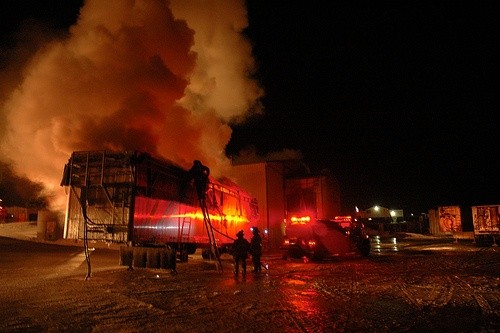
[283,216,324,257]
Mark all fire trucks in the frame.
[133,171,265,259]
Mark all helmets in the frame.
[249,227,258,233]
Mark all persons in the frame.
[232,231,251,281]
[249,226,263,273]
[188,159,210,206]
